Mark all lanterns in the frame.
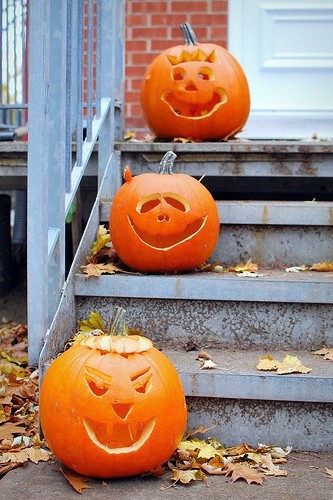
[141,22,252,143]
[38,307,188,479]
[109,151,220,272]
